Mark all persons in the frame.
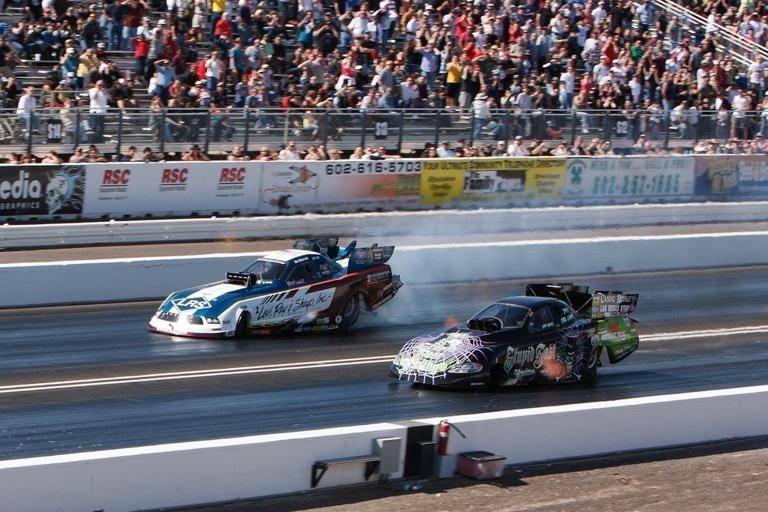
[1,0,768,167]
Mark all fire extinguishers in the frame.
[437,419,466,455]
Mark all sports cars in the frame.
[141,234,402,342]
[385,278,643,391]
